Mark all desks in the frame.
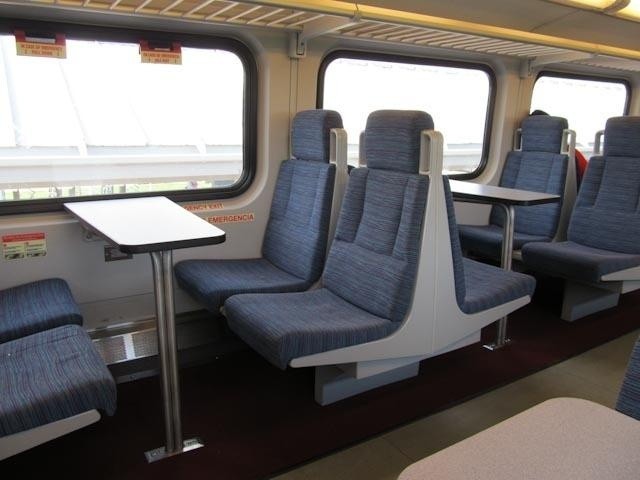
[62,193,228,463]
[445,175,562,354]
[396,394,640,480]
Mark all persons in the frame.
[532,109,588,176]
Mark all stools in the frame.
[0,324,121,480]
[0,277,84,346]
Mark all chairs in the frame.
[611,330,640,424]
[434,174,537,351]
[171,109,351,311]
[222,109,440,410]
[520,115,640,322]
[455,113,578,279]
